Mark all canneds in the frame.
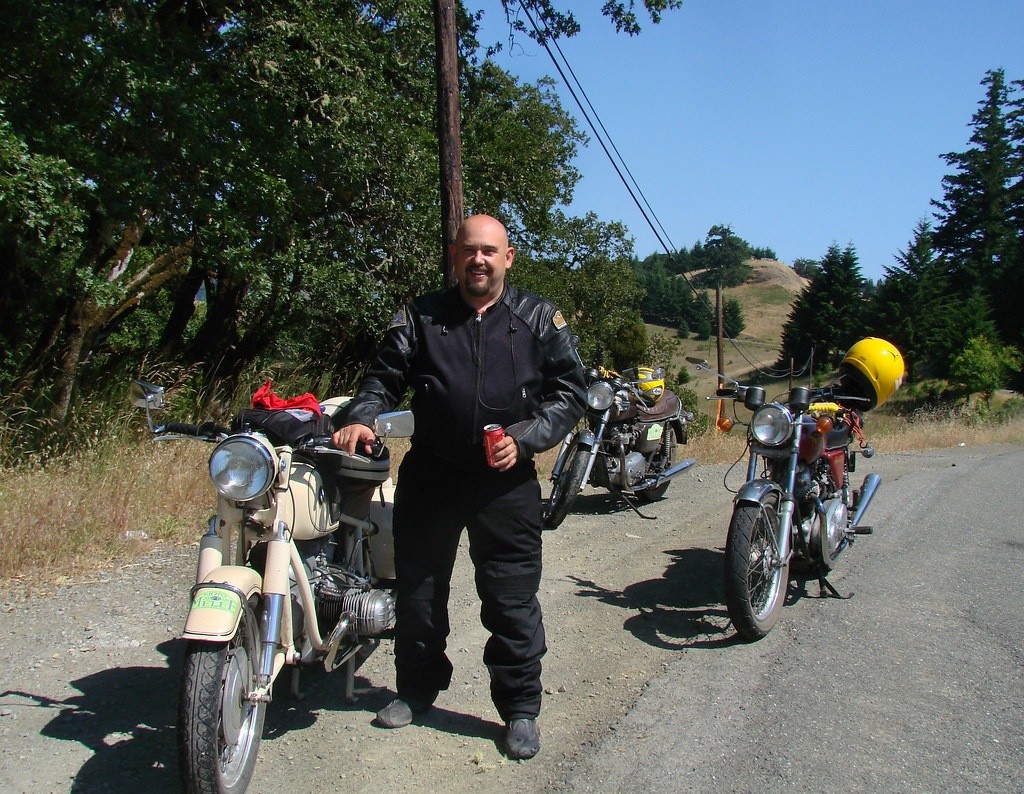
[483,424,506,468]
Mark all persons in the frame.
[334,214,589,760]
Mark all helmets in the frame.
[624,367,665,403]
[839,338,904,412]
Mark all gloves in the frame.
[231,408,313,445]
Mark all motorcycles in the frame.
[545,335,697,530]
[128,379,415,794]
[682,355,882,642]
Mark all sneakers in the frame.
[375,691,440,727]
[504,718,542,759]
